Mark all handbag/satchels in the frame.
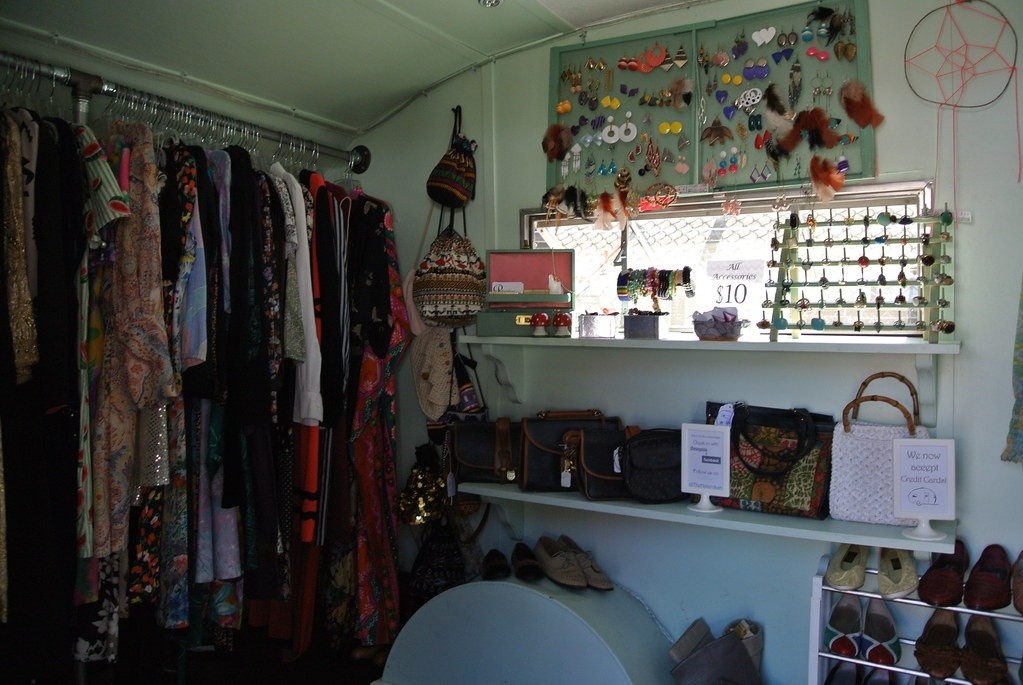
[520,410,603,492]
[443,416,520,484]
[617,428,698,505]
[829,370,930,526]
[705,400,834,521]
[410,324,461,420]
[581,424,648,501]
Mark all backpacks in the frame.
[402,200,440,334]
[422,323,487,442]
[398,442,452,542]
[413,199,486,328]
[406,518,472,617]
[426,105,477,209]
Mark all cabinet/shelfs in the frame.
[457,333,962,554]
[808,556,1023,685]
[758,202,953,343]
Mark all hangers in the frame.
[0,50,58,148]
[88,76,360,195]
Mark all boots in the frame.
[671,632,763,685]
[669,618,716,665]
[722,618,763,677]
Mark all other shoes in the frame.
[510,542,546,583]
[557,534,615,590]
[822,541,1023,685]
[534,535,590,590]
[479,546,511,581]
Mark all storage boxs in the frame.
[623,313,669,339]
[475,247,576,336]
[579,315,619,337]
